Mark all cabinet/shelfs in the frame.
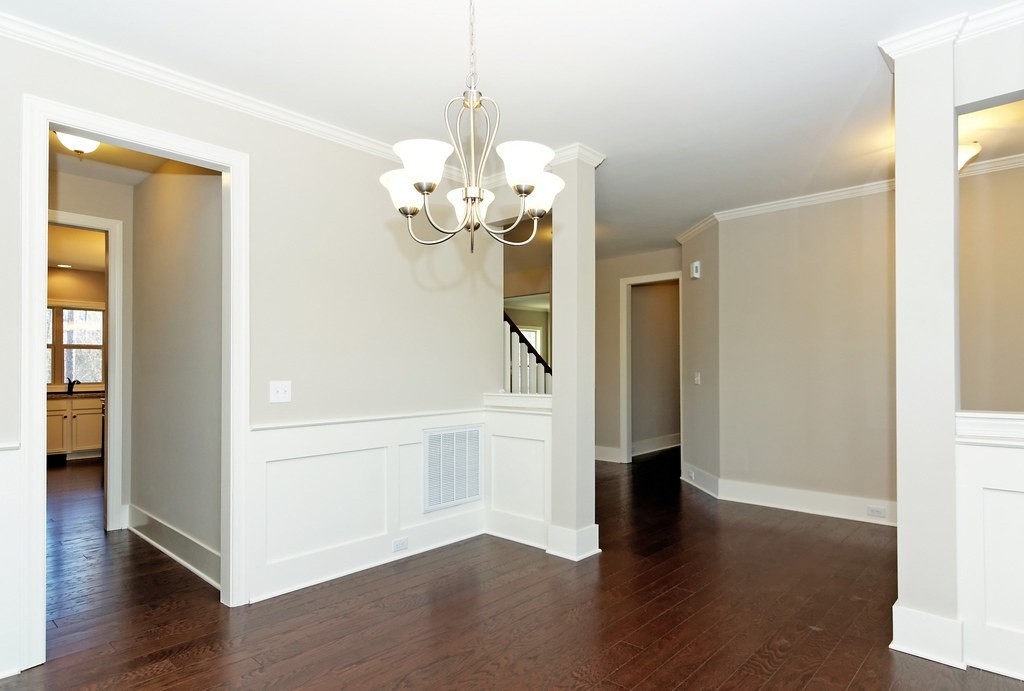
[46,398,101,454]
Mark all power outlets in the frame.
[867,505,885,517]
[687,470,694,481]
[391,537,408,554]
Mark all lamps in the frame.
[54,131,100,155]
[374,0,567,254]
